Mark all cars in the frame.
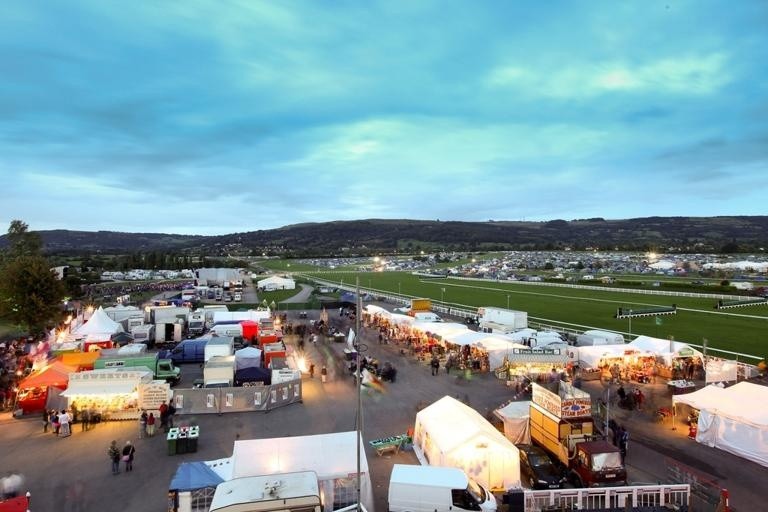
[514,443,568,489]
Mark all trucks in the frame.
[188,311,205,336]
[155,318,185,349]
[93,356,181,387]
[474,306,528,332]
[530,380,628,488]
[206,279,244,302]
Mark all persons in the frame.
[39,399,105,438]
[140,398,177,439]
[107,439,120,474]
[122,441,136,471]
[0,469,26,499]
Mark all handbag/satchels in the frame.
[121,455,130,462]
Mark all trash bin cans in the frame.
[333,331,346,343]
[166,425,199,456]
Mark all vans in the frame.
[167,339,207,364]
[387,463,498,512]
